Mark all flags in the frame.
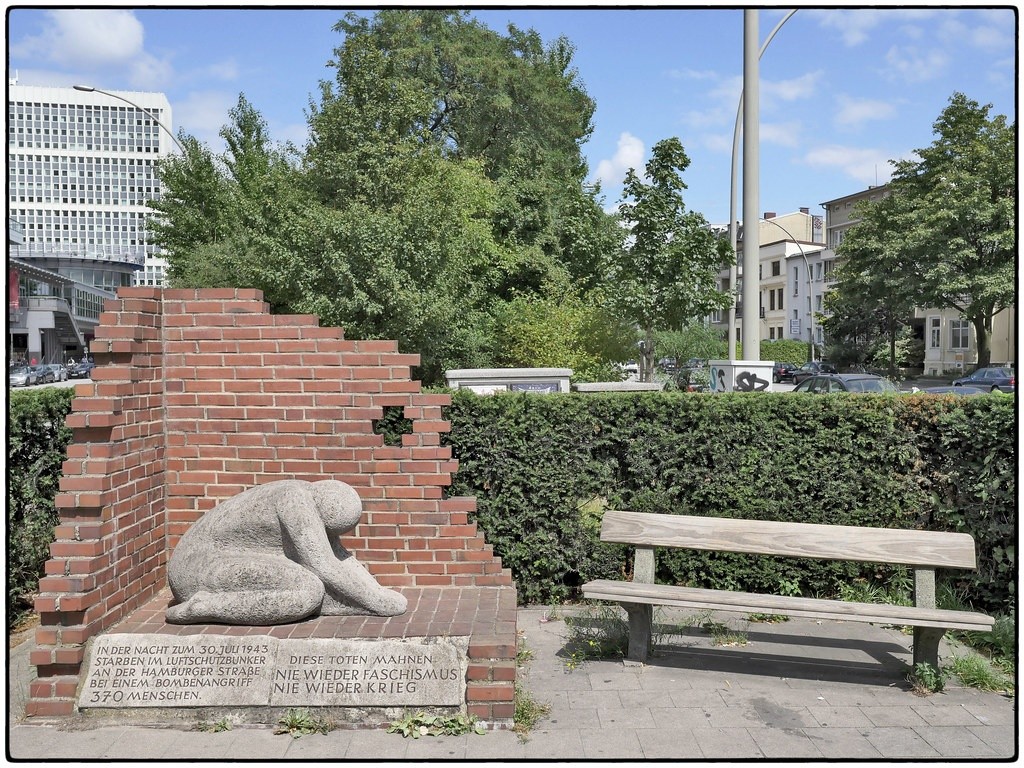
[10,268,19,309]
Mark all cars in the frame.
[617,358,639,374]
[791,361,844,386]
[909,387,990,395]
[773,361,799,384]
[676,366,710,393]
[10,363,69,388]
[658,356,710,375]
[70,363,94,379]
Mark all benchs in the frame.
[582,511,995,692]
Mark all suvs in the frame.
[953,366,1015,393]
[782,371,901,394]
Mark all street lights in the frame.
[73,84,191,165]
[726,215,817,361]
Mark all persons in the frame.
[81,356,89,364]
[21,358,29,367]
[31,357,37,365]
[68,356,75,365]
[165,478,407,626]
[10,358,15,373]
